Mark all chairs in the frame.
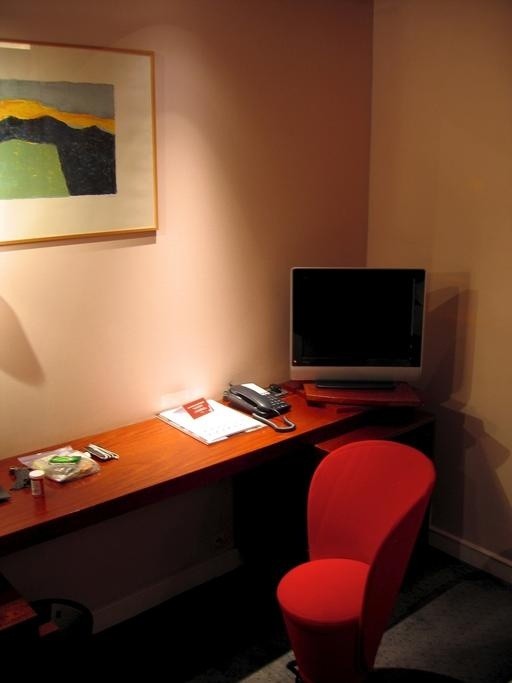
[276,439,436,683]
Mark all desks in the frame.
[0,378,440,558]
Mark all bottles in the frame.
[29,469,46,498]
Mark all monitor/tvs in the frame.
[290,266,427,390]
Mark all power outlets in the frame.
[211,531,225,546]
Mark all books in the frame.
[158,398,266,445]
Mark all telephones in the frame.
[225,383,290,419]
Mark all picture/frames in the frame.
[0,38,160,246]
